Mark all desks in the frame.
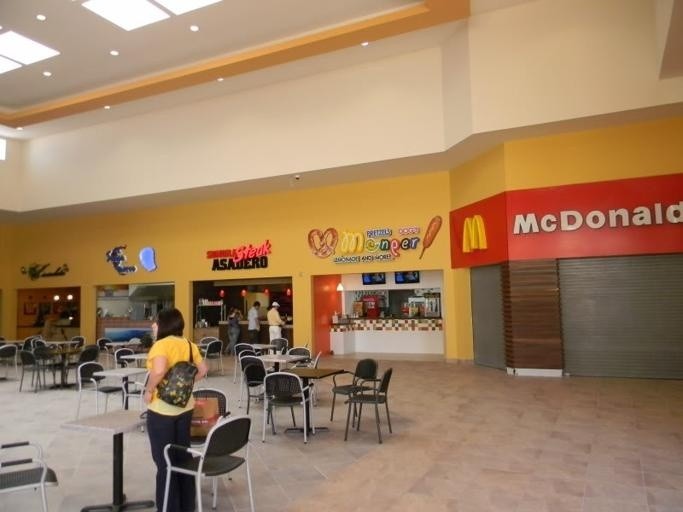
[285,368,344,435]
[120,353,149,360]
[252,343,276,355]
[257,354,310,373]
[57,408,154,511]
[39,349,81,389]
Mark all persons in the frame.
[247,302,261,345]
[221,306,243,355]
[142,307,208,512]
[266,301,285,354]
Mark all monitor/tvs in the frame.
[395,272,419,284]
[362,273,386,284]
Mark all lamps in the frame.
[335,273,344,292]
[218,283,292,299]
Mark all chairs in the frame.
[262,371,315,446]
[343,367,393,444]
[160,413,254,511]
[330,359,378,428]
[202,337,224,377]
[114,349,137,369]
[0,439,59,511]
[98,338,142,368]
[76,362,123,415]
[233,342,262,384]
[238,347,321,416]
[183,386,227,509]
[0,335,87,393]
[270,338,288,354]
[122,373,149,409]
[92,366,148,410]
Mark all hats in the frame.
[272,302,280,307]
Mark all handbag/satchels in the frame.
[156,340,198,408]
